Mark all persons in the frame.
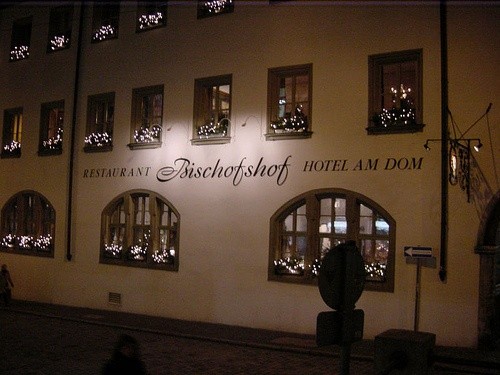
[102,333,148,375]
